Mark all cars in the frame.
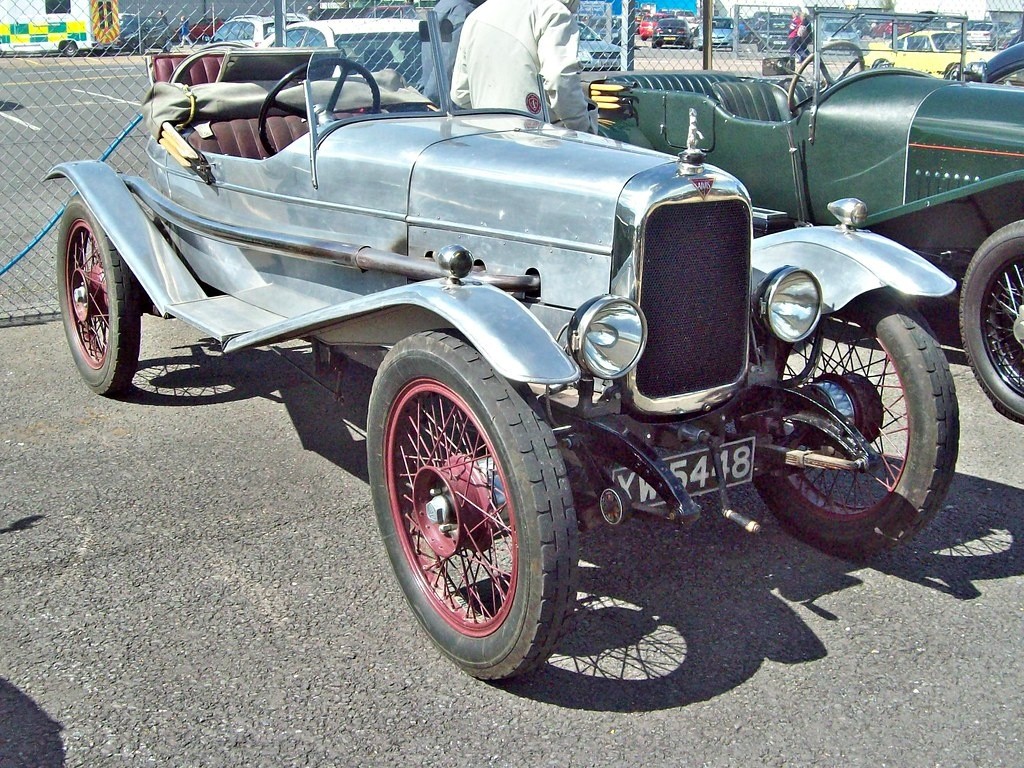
[255,19,424,89]
[870,22,910,38]
[640,13,677,41]
[593,16,622,46]
[757,15,793,52]
[577,21,621,71]
[739,18,756,44]
[672,10,696,23]
[41,43,961,680]
[863,30,999,80]
[698,17,734,51]
[587,10,1024,424]
[821,19,861,48]
[652,18,693,49]
[966,21,1024,49]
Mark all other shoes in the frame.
[797,61,802,64]
[810,61,813,65]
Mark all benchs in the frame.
[153,54,231,84]
[189,101,428,161]
[606,73,740,101]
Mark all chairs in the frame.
[362,50,404,74]
[709,80,801,124]
[777,79,819,107]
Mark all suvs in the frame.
[202,13,309,48]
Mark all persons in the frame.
[785,6,815,64]
[421,0,486,106]
[449,0,602,136]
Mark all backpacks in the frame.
[797,25,807,37]
